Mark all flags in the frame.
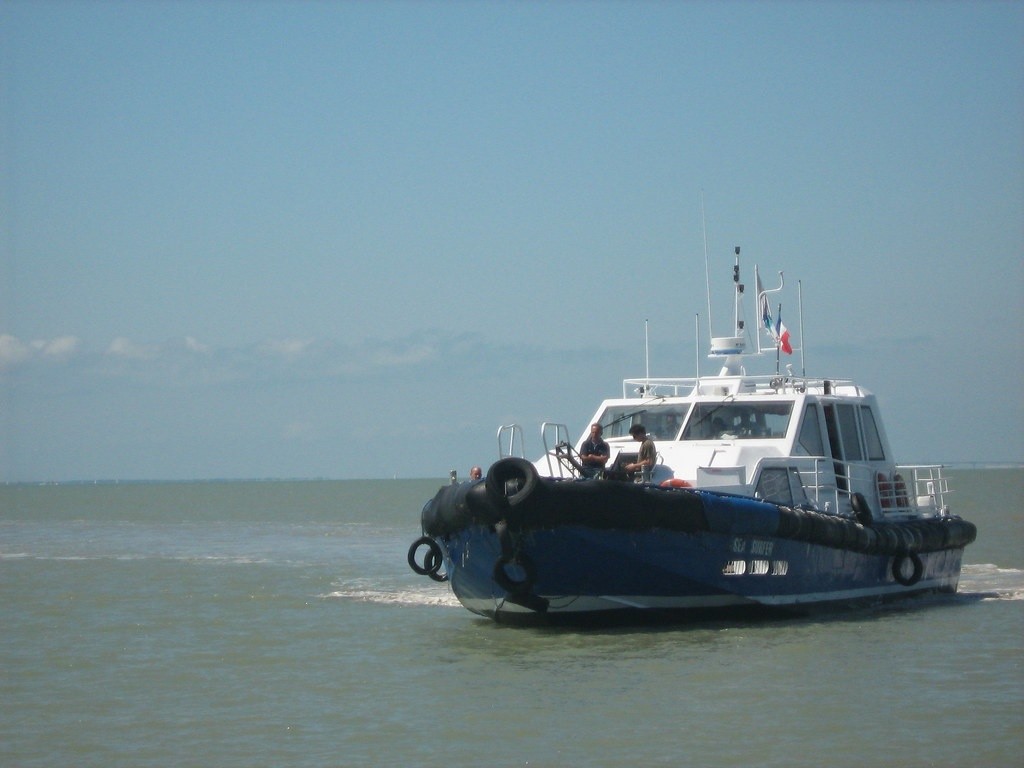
[775,318,791,355]
[758,272,779,343]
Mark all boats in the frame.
[420,215,978,630]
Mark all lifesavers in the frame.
[891,551,923,586]
[407,537,442,575]
[850,492,873,526]
[878,472,892,506]
[484,455,541,511]
[424,548,450,582]
[894,474,909,507]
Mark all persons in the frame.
[580,423,610,479]
[735,413,758,433]
[470,466,482,482]
[705,417,726,439]
[624,425,656,482]
